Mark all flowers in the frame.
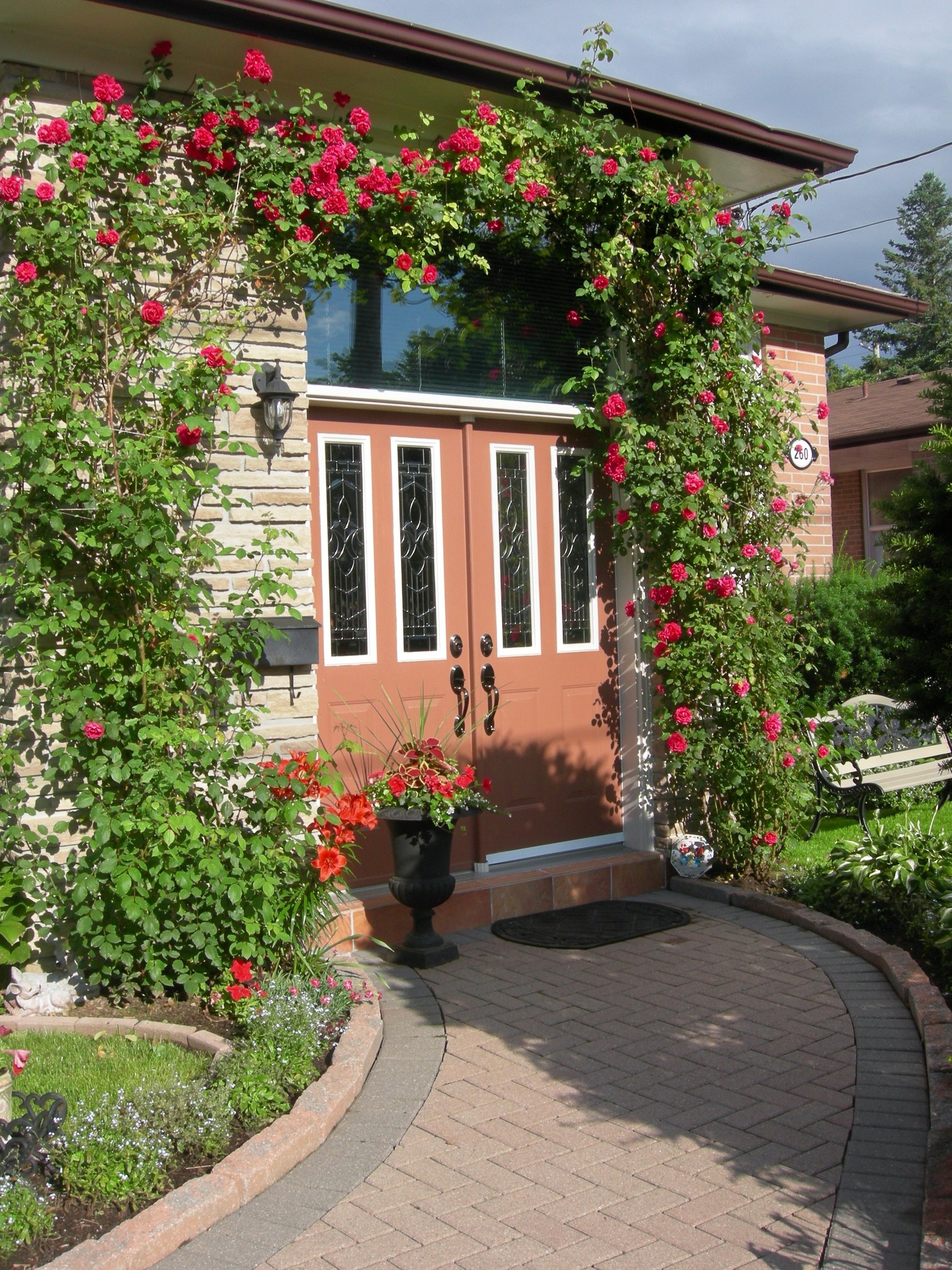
[324,682,513,834]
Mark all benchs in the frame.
[808,692,952,838]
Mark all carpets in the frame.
[492,900,692,949]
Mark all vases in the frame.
[372,807,463,969]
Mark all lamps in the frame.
[252,358,300,457]
